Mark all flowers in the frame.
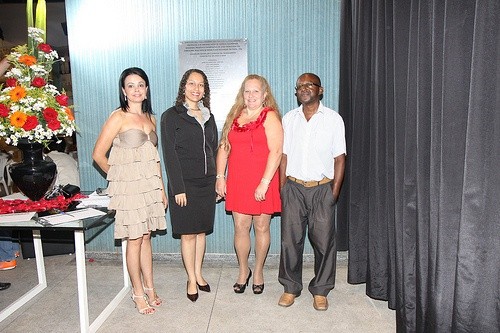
[0,27,82,147]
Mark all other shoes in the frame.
[187,280,198,302]
[0,282,11,291]
[196,281,210,292]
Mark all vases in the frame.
[5,138,57,200]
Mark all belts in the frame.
[289,176,332,188]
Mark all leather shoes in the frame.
[313,295,328,310]
[278,291,301,307]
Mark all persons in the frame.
[160,69,220,305]
[93,68,169,315]
[277,73,346,311]
[0,136,81,292]
[214,75,283,294]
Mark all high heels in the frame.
[233,267,252,293]
[143,285,162,307]
[131,288,154,314]
[252,282,264,294]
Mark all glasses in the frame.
[295,82,319,90]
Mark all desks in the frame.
[0,191,132,333]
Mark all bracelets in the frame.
[216,175,225,178]
[261,178,271,186]
